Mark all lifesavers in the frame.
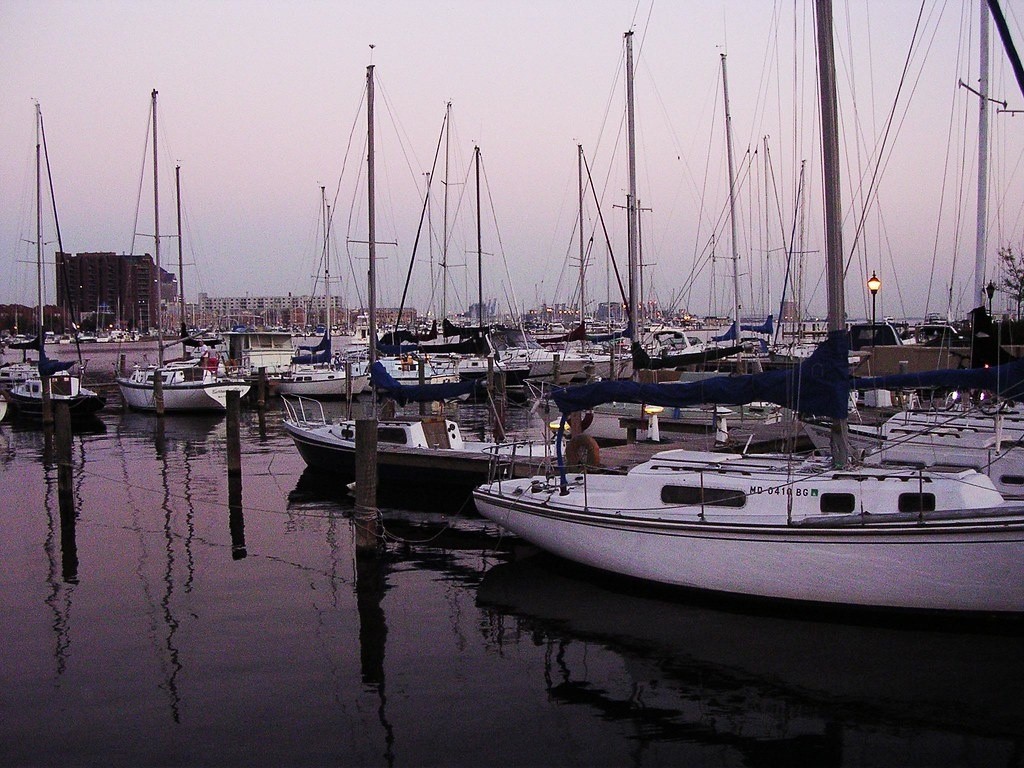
[565,433,601,469]
[226,358,237,372]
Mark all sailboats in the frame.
[1,0,1024,612]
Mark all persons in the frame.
[200,340,208,367]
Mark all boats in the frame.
[474,555,1024,765]
